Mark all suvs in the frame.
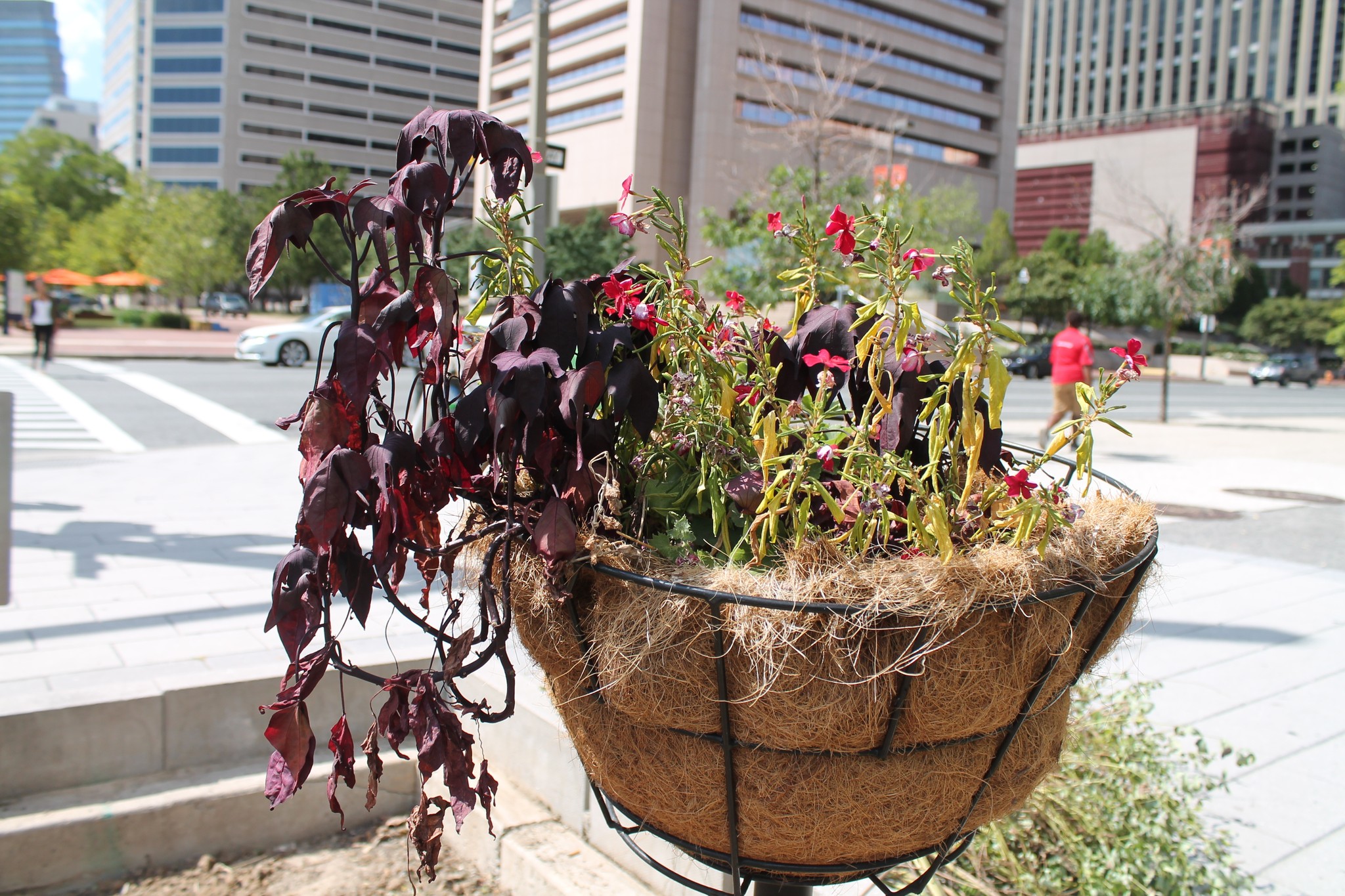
[1252,353,1321,386]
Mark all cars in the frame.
[234,305,426,368]
[1001,341,1053,379]
[205,292,249,316]
[62,294,103,312]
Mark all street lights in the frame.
[1016,264,1031,336]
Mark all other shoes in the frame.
[1038,429,1049,449]
[1070,444,1080,451]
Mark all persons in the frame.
[25,276,56,370]
[1036,312,1095,452]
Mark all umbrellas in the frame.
[0,267,162,288]
